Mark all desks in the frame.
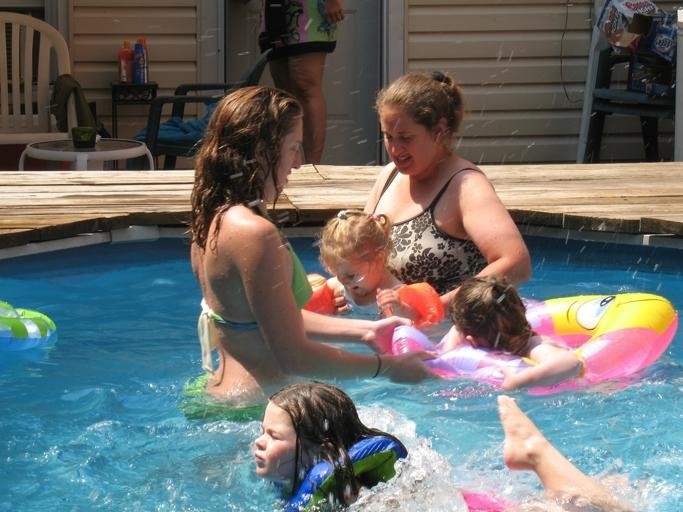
[18,137,155,171]
[675,6,683,161]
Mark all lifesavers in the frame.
[391,292,678,397]
[303,273,445,330]
[0,300,57,351]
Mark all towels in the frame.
[48,74,111,138]
[125,103,222,170]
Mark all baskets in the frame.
[112,84,159,101]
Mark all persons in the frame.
[191,87,440,382]
[256,0,345,165]
[330,69,532,313]
[449,275,582,392]
[498,395,594,492]
[254,384,368,506]
[320,209,420,324]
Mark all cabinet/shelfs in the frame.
[109,79,158,169]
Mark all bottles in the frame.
[117,38,146,83]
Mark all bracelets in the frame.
[373,354,382,378]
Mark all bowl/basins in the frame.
[71,125,98,147]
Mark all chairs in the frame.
[0,10,78,171]
[142,45,274,170]
[577,0,683,163]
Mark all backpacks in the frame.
[595,0,677,99]
